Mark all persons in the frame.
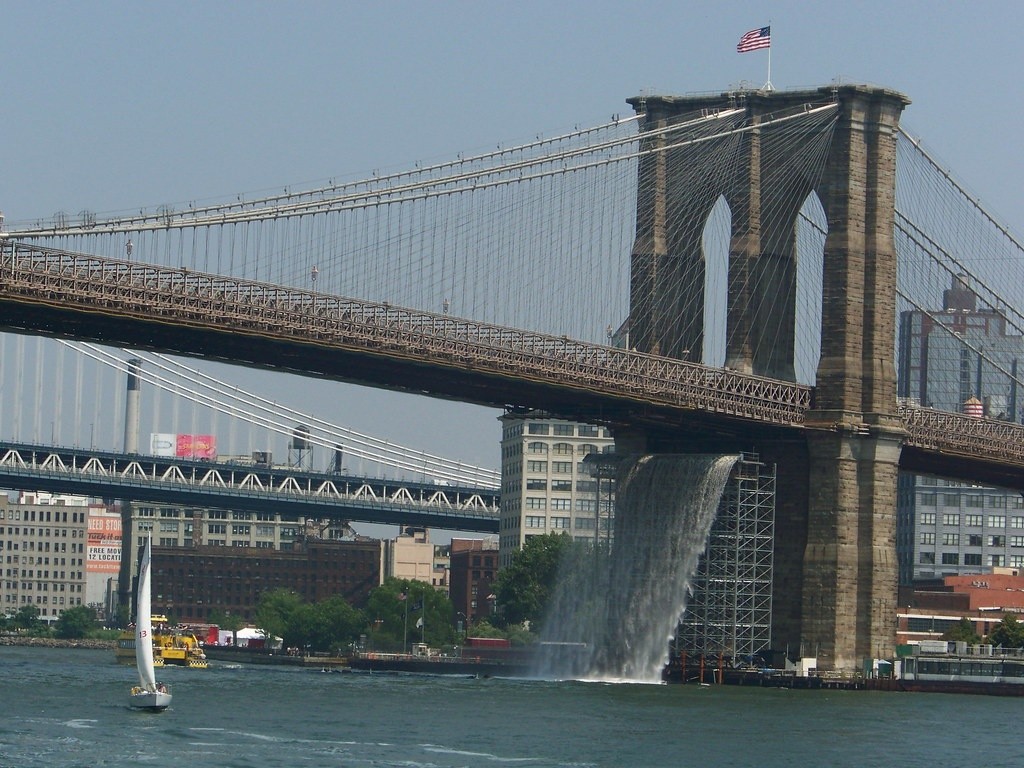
[157,681,167,693]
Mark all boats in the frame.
[115,614,208,669]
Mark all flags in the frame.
[411,599,423,612]
[416,617,423,628]
[736,25,770,53]
[401,611,406,624]
[400,585,407,601]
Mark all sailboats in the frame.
[127,531,173,707]
